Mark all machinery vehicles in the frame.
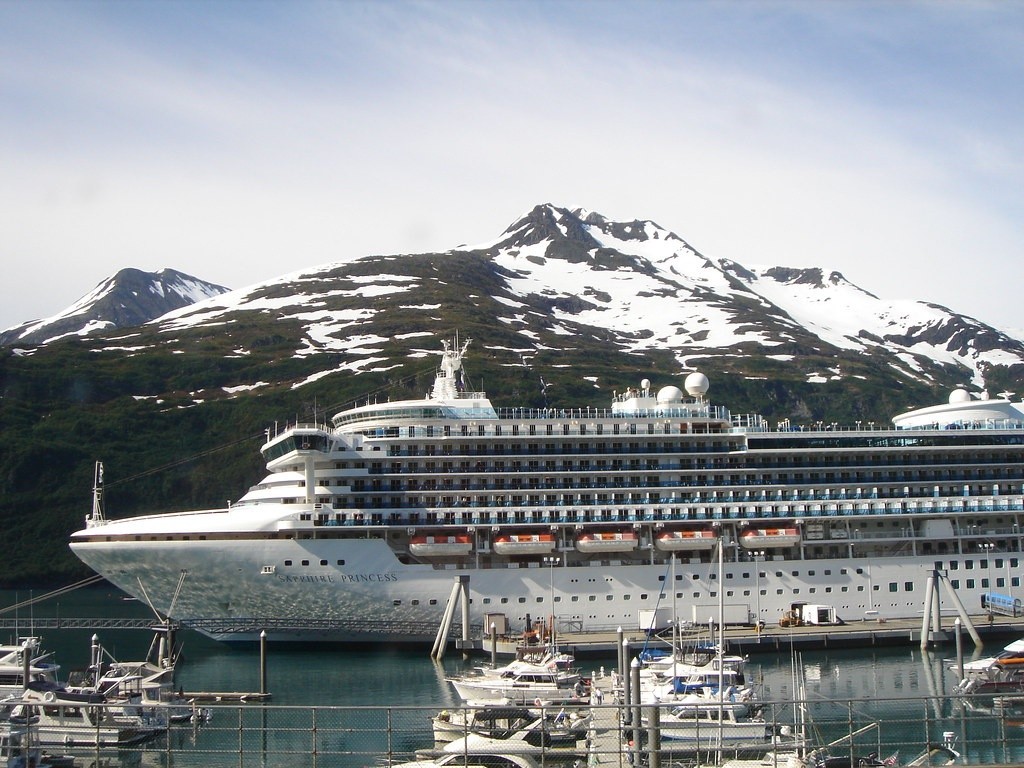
[778,610,805,627]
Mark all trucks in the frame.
[692,605,766,628]
[638,607,680,637]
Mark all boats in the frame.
[575,532,638,552]
[629,539,960,768]
[950,638,1024,686]
[492,535,556,555]
[0,681,169,754]
[392,654,588,768]
[0,636,67,708]
[62,662,208,728]
[738,528,800,547]
[654,531,717,550]
[409,534,473,555]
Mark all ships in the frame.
[69,339,1023,644]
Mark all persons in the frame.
[595,689,604,705]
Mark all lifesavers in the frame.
[43,691,56,702]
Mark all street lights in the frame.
[260,630,268,693]
[748,543,996,621]
[490,622,497,669]
[544,556,561,645]
[91,633,99,687]
